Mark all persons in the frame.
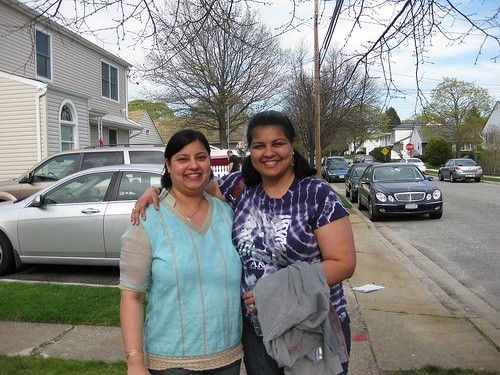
[226,150,239,172]
[118,130,243,375]
[133,109,355,375]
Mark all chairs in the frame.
[116,175,140,201]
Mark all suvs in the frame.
[0,143,166,203]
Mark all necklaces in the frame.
[185,202,203,222]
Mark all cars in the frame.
[322,155,374,183]
[357,162,443,222]
[437,158,483,183]
[344,163,379,203]
[0,163,167,277]
[399,158,427,174]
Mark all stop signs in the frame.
[405,143,414,152]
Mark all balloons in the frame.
[236,141,248,157]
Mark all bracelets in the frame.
[125,349,144,358]
